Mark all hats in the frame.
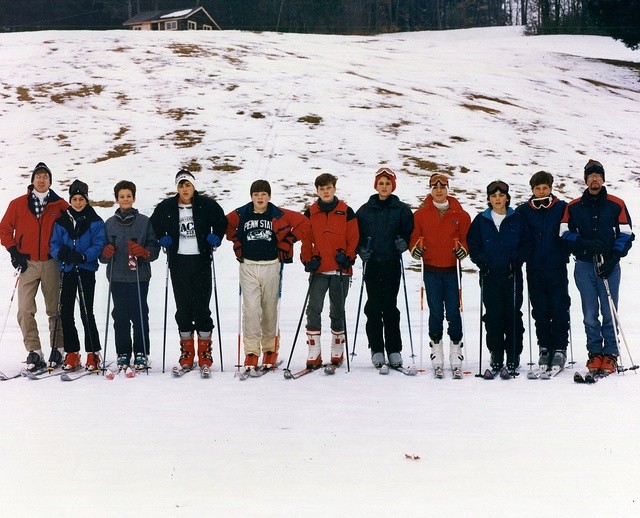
[584,159,605,183]
[374,172,396,192]
[69,180,89,204]
[175,169,195,189]
[31,162,52,184]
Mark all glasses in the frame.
[375,166,396,179]
[36,175,50,181]
[431,174,449,184]
[529,193,554,209]
[486,181,509,193]
[178,206,193,208]
[114,207,138,226]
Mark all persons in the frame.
[514,171,570,369]
[49,180,107,371]
[300,174,359,368]
[407,174,470,369]
[465,182,523,371]
[357,169,413,366]
[151,171,228,368]
[0,163,69,370]
[225,181,310,368]
[557,159,634,373]
[103,182,160,368]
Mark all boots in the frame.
[305,331,322,367]
[197,337,213,368]
[330,329,345,364]
[179,338,195,368]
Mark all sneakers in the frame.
[244,354,259,370]
[387,351,403,367]
[60,352,81,370]
[448,340,464,369]
[489,351,504,369]
[587,353,603,373]
[600,354,617,373]
[26,351,45,370]
[538,347,553,365]
[263,351,278,366]
[116,352,132,369]
[85,351,101,370]
[370,350,385,367]
[429,340,444,369]
[47,347,65,368]
[507,353,520,370]
[133,352,148,370]
[551,349,567,366]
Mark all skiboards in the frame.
[527,361,575,379]
[283,358,344,379]
[106,359,152,380]
[27,360,116,381]
[236,360,284,380]
[574,364,625,383]
[172,362,211,378]
[375,361,417,375]
[484,364,522,379]
[435,368,463,378]
[0,361,65,380]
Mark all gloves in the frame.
[102,243,115,259]
[584,237,606,255]
[277,231,297,259]
[58,245,71,261]
[476,260,489,271]
[411,245,426,260]
[131,243,151,259]
[358,246,373,263]
[233,240,243,259]
[453,242,468,261]
[395,238,407,252]
[67,252,87,265]
[158,235,172,248]
[336,254,351,269]
[207,234,220,247]
[9,246,27,272]
[304,261,320,271]
[596,255,617,278]
